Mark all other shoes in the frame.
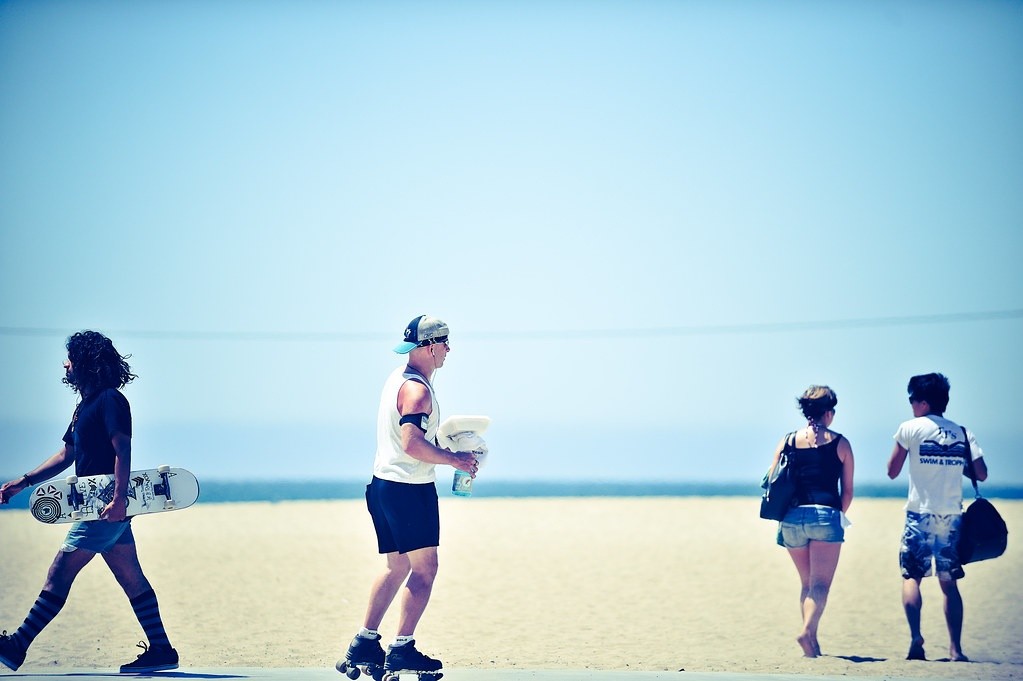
[120,641,179,673]
[0,630,26,671]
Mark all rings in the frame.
[475,460,479,465]
[471,468,476,473]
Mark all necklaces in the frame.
[71,400,85,433]
[407,364,429,381]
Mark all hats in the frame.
[393,314,449,354]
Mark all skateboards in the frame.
[28,465,200,525]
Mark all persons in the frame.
[887,372,987,661]
[336,314,479,681]
[770,386,854,657]
[0,332,180,674]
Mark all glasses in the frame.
[909,396,922,404]
[435,341,449,346]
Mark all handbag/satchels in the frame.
[959,498,1008,565]
[759,431,801,521]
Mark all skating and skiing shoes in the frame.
[336,632,387,681]
[382,640,443,681]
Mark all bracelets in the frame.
[24,474,34,486]
